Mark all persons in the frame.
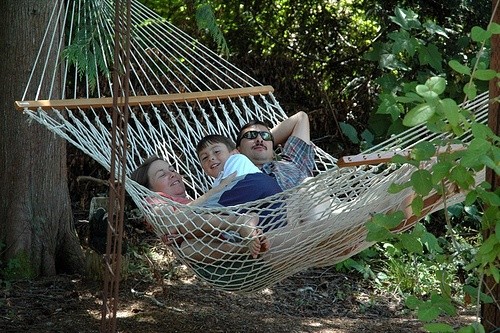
[181,134,283,263]
[130,155,416,293]
[236,111,415,237]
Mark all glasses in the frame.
[239,130,273,144]
